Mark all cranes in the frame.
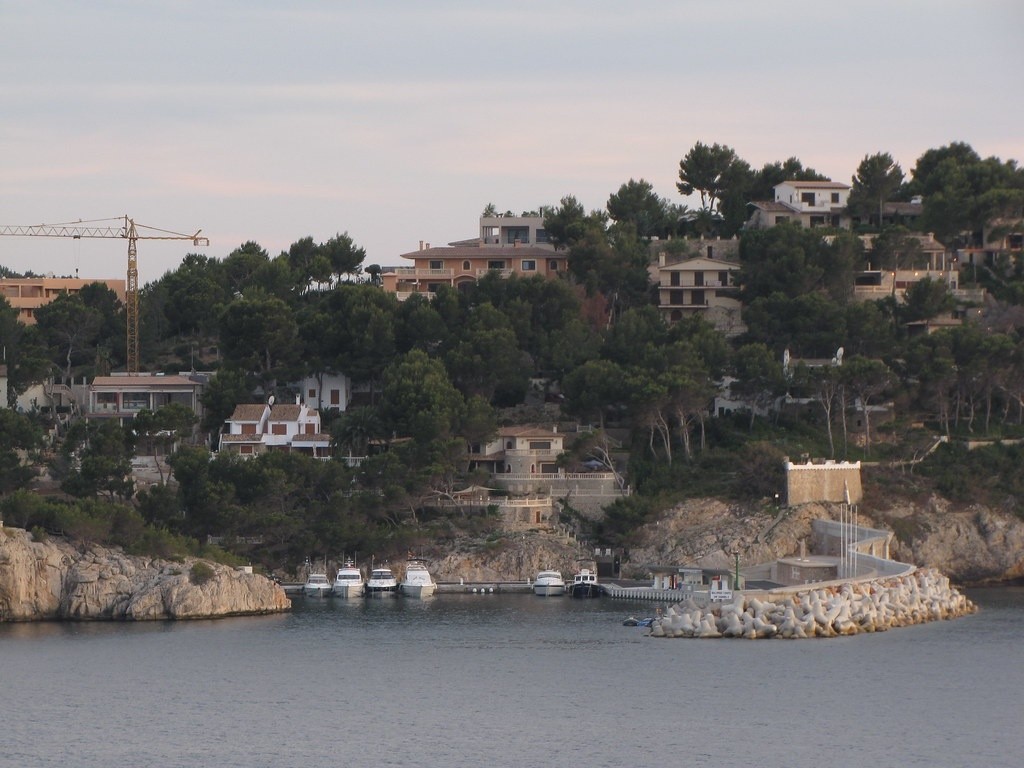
[2,212,211,377]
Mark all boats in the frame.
[334,549,364,599]
[399,560,438,599]
[532,568,566,597]
[569,568,606,596]
[305,573,331,598]
[367,564,397,593]
